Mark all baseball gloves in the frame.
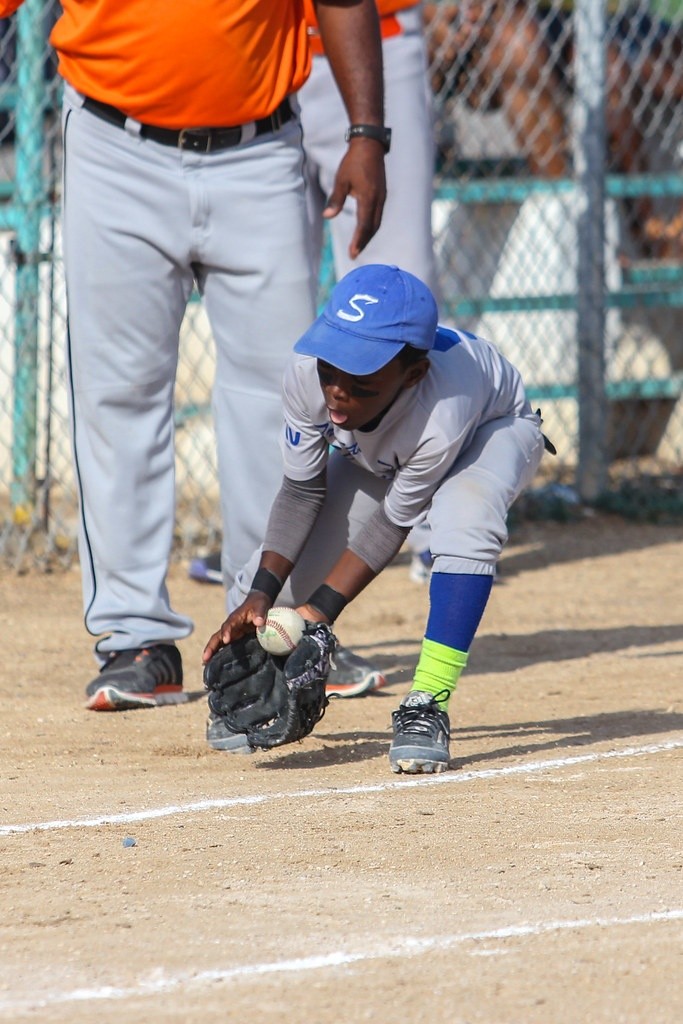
[202,616,337,755]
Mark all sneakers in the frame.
[85,644,188,711]
[325,639,387,698]
[389,690,451,773]
[206,711,249,750]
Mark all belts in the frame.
[83,96,298,154]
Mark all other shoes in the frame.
[188,550,223,583]
[409,550,502,585]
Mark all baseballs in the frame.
[254,605,308,658]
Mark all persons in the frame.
[0,0,682,717]
[200,264,546,775]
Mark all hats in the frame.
[294,264,438,377]
